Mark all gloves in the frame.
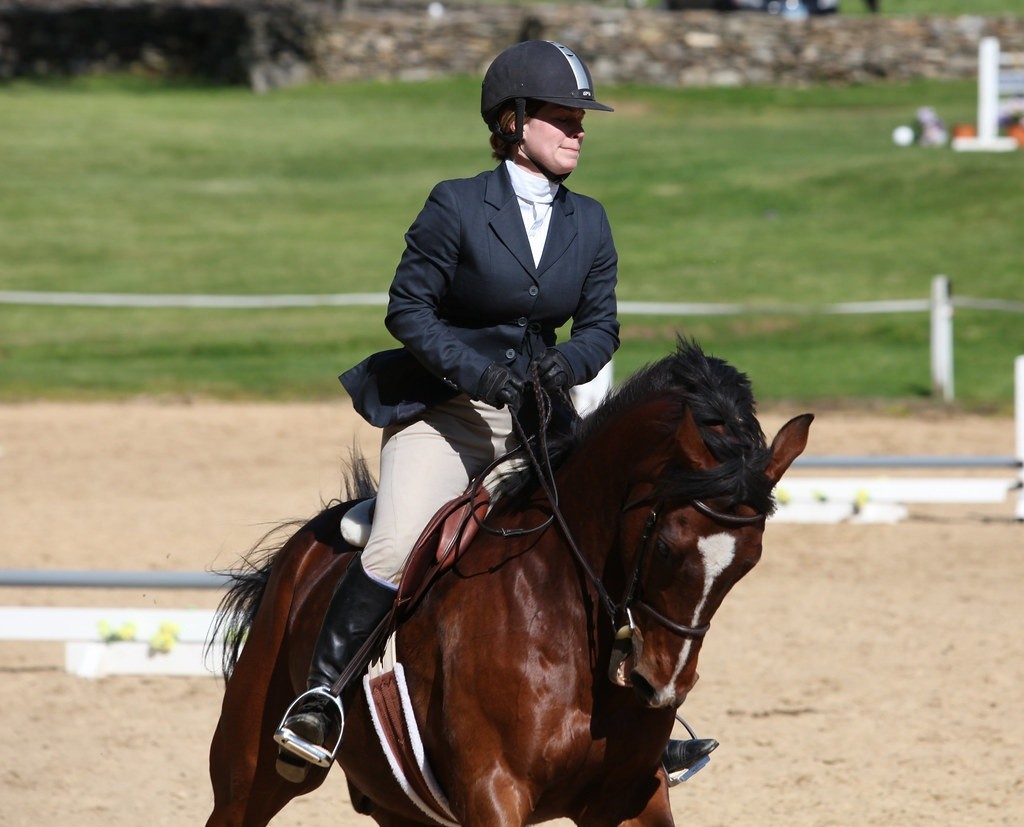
[475,363,524,410]
[534,349,574,391]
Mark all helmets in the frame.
[479,40,615,115]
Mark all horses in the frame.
[201,346,815,826]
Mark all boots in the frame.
[660,736,719,774]
[275,552,398,783]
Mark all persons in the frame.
[274,38,720,786]
